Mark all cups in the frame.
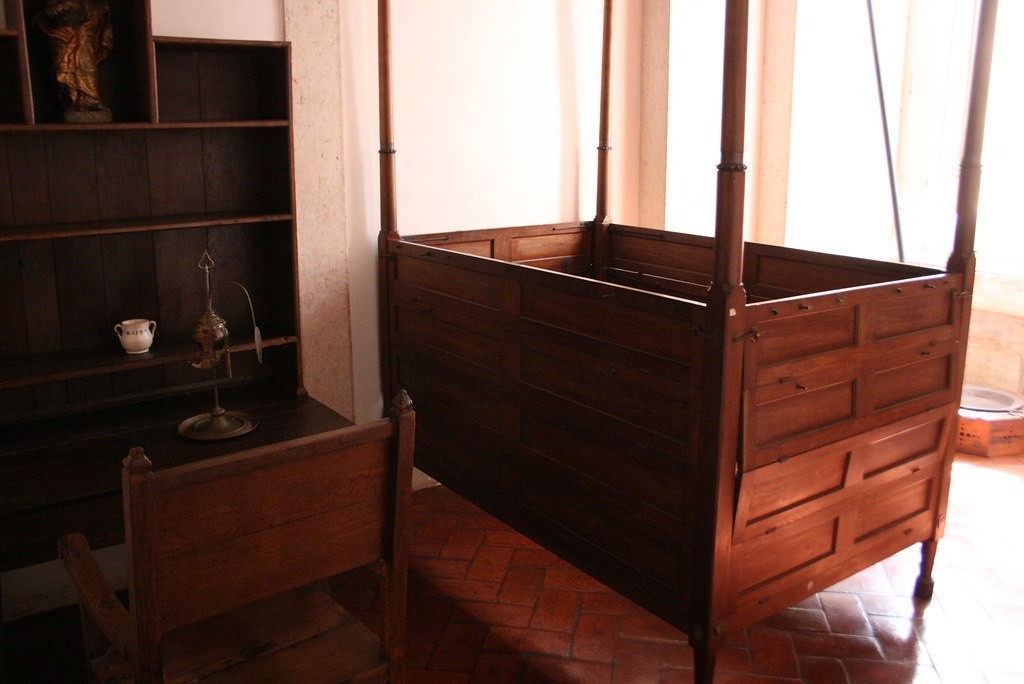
[114,319,156,354]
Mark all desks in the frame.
[0,380,356,572]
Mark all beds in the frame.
[378,2,993,684]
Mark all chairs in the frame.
[58,389,418,684]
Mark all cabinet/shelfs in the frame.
[0,0,310,404]
